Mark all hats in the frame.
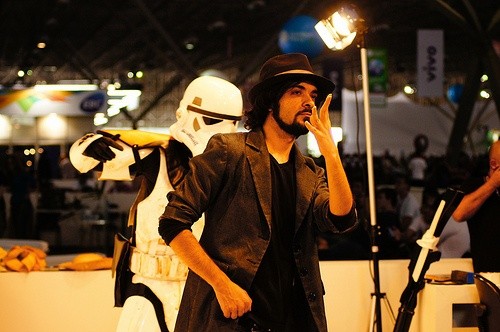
[247,53,335,107]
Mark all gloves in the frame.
[79,134,124,164]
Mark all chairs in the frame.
[474,274,500,332]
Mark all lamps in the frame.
[314,2,395,332]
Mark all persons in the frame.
[0,135,500,259]
[70,75,243,332]
[158,54,358,332]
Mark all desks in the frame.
[420,283,481,332]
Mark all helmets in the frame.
[170,76,243,157]
